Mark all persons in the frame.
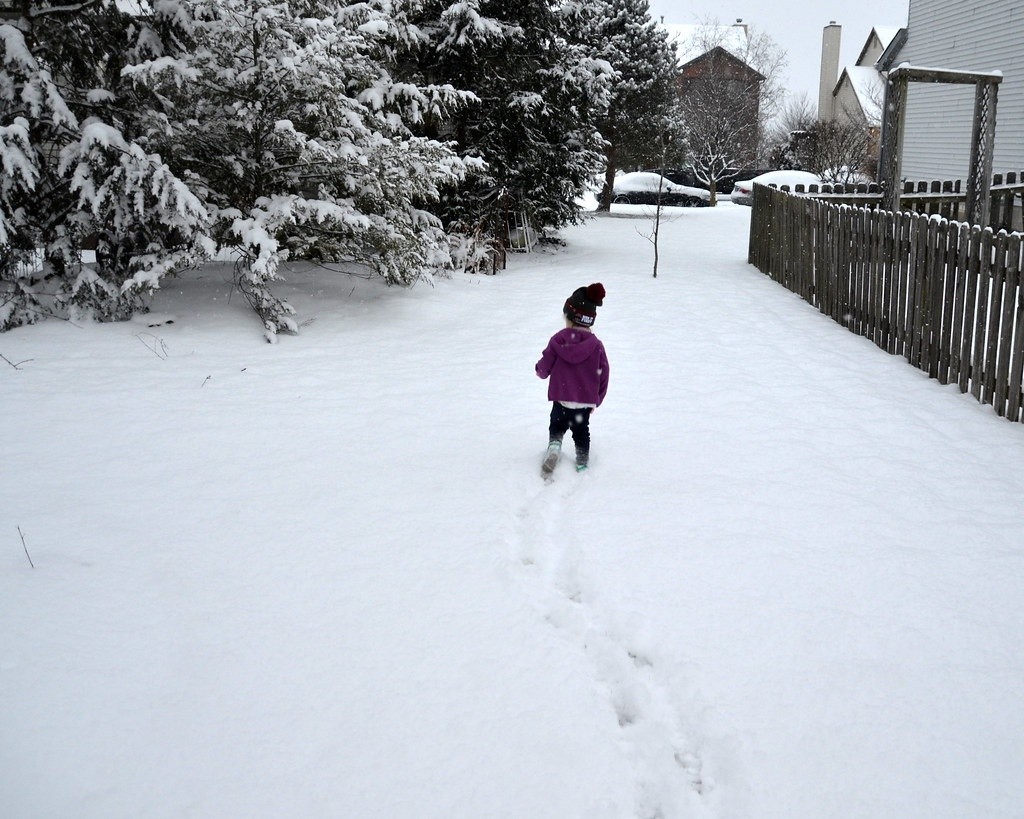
[535,283,610,474]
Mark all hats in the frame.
[563,282,605,327]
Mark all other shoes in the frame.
[575,464,590,473]
[541,446,559,480]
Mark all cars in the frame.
[593,172,711,207]
[731,170,834,207]
